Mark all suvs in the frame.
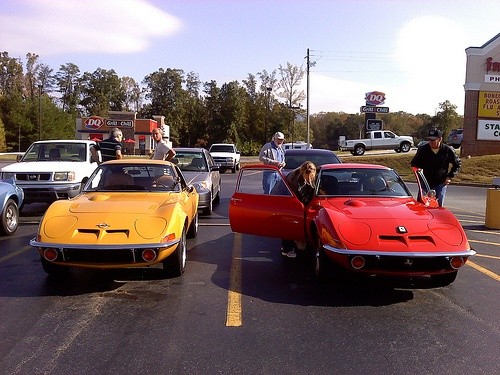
[209,143,242,173]
[447,128,464,149]
[0,139,103,212]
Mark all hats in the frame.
[275,132,284,139]
[425,128,442,139]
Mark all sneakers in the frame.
[282,250,296,257]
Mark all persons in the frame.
[89,128,124,170]
[258,132,286,194]
[270,161,327,257]
[409,129,460,207]
[150,128,176,175]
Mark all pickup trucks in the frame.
[340,130,415,156]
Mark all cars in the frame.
[1,178,26,236]
[280,148,355,184]
[140,147,221,216]
[228,162,477,284]
[29,158,201,277]
